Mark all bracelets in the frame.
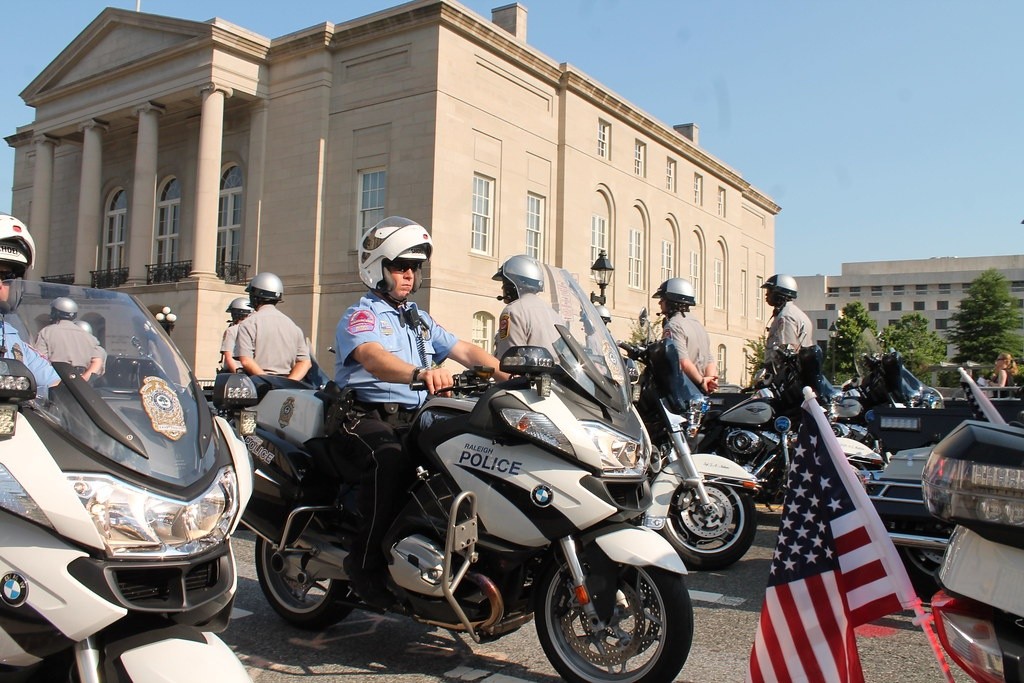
[988,380,992,384]
[413,367,421,379]
[698,376,705,386]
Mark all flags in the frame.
[957,374,1007,426]
[749,407,913,683]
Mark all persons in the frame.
[760,273,813,394]
[487,254,571,374]
[0,212,63,389]
[985,352,1019,399]
[651,277,720,432]
[232,272,313,381]
[36,295,112,408]
[334,216,510,563]
[218,297,253,375]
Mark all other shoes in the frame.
[342,555,384,603]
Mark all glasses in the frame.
[387,262,419,274]
[0,269,22,286]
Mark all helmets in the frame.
[0,211,36,272]
[48,297,78,323]
[357,215,434,293]
[491,254,544,293]
[244,272,283,302]
[651,277,696,306]
[74,320,92,335]
[225,297,252,315]
[759,275,798,300]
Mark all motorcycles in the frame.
[0,278,257,682]
[212,255,695,683]
[614,328,1024,683]
